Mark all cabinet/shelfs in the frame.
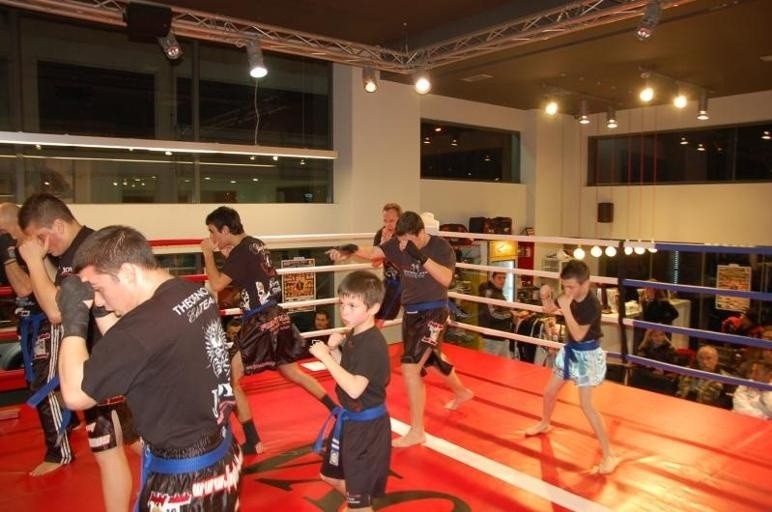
[543,257,570,296]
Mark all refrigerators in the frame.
[444,234,520,356]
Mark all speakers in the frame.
[122,1,173,45]
[597,202,614,223]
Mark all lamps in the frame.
[245,40,268,79]
[638,66,715,121]
[633,2,663,42]
[155,32,183,61]
[573,239,657,262]
[411,67,433,96]
[361,63,379,94]
[536,82,619,129]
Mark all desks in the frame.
[601,298,692,357]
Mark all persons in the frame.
[524,261,621,475]
[201,207,339,455]
[628,280,772,416]
[311,269,391,512]
[478,272,516,357]
[0,203,81,477]
[325,212,474,448]
[373,204,403,331]
[227,317,243,346]
[19,194,142,512]
[307,311,330,350]
[56,225,243,512]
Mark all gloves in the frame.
[0,233,18,263]
[55,277,95,339]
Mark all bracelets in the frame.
[5,259,17,265]
